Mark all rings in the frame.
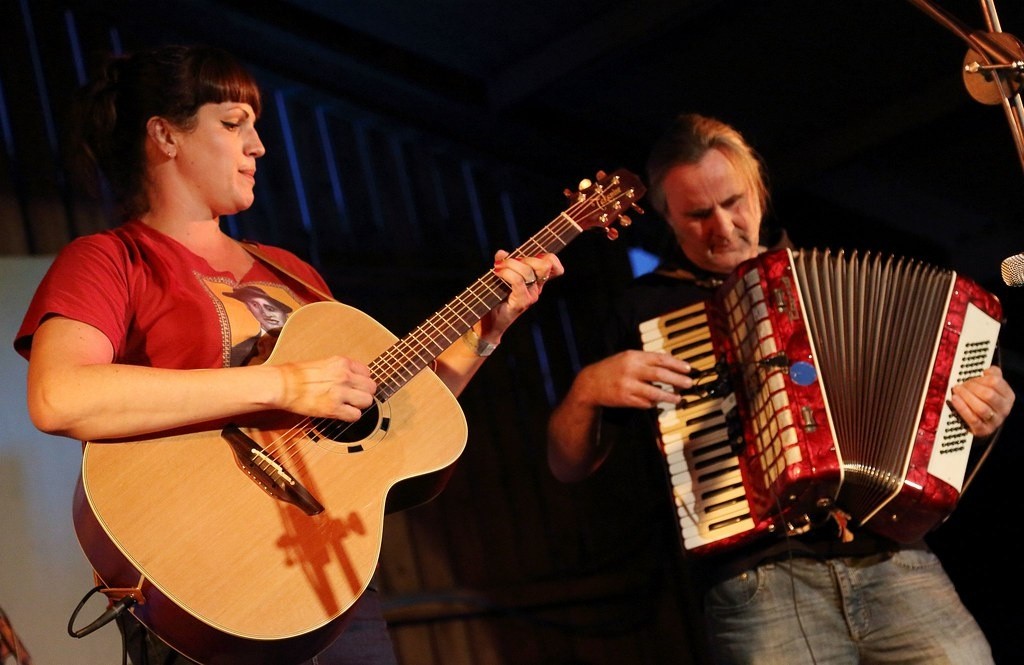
[525,277,538,286]
[985,411,995,421]
[538,275,549,281]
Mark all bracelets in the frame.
[461,326,501,356]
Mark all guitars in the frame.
[71,165,648,665]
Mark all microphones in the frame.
[1000,252,1024,287]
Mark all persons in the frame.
[549,113,1017,665]
[14,38,565,665]
